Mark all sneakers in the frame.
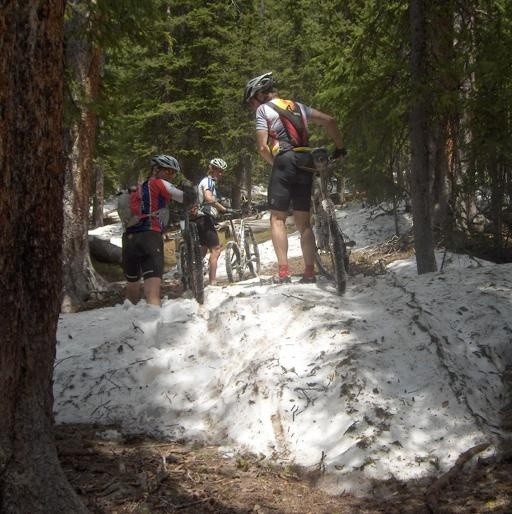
[260,276,291,283]
[299,276,317,283]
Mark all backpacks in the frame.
[118,187,147,227]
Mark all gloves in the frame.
[176,179,186,188]
[226,208,242,215]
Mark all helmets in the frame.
[245,72,275,103]
[150,154,180,172]
[209,157,227,169]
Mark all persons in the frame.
[193,157,239,286]
[243,71,348,285]
[121,153,196,309]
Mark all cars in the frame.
[240,186,261,194]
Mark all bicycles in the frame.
[152,196,227,308]
[211,194,266,282]
[295,143,361,294]
